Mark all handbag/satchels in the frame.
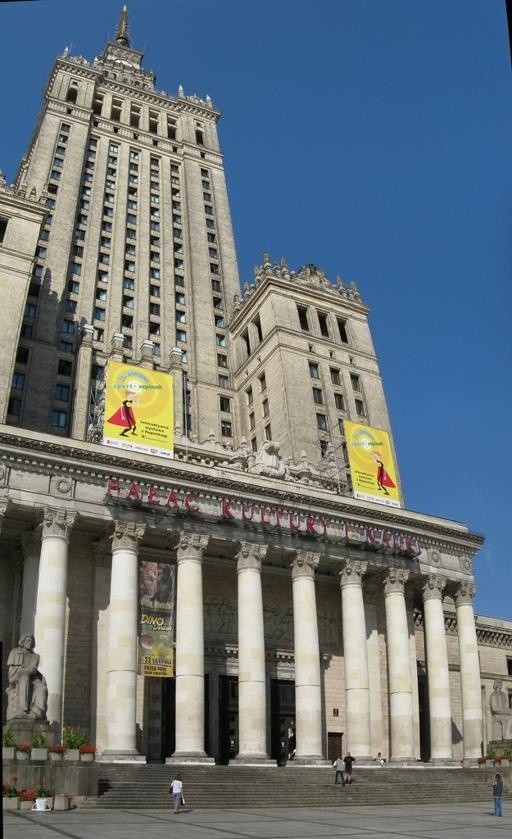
[169,788,172,793]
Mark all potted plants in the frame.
[475,745,511,769]
[1,719,97,765]
[3,780,73,814]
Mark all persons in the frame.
[333,755,346,787]
[6,634,48,720]
[373,452,390,495]
[119,390,138,437]
[376,752,385,765]
[343,752,355,783]
[493,774,503,816]
[491,679,512,740]
[171,774,183,815]
